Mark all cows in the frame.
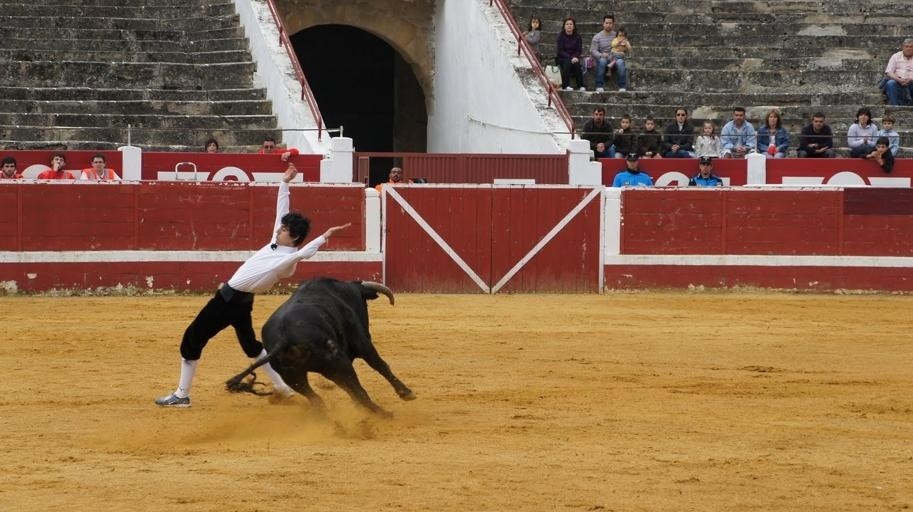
[224,276,416,434]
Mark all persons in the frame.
[580,107,789,189]
[153,164,353,410]
[376,167,413,198]
[0,155,25,179]
[878,39,913,107]
[522,15,543,63]
[203,137,220,153]
[589,15,632,91]
[555,18,587,91]
[37,152,78,180]
[253,136,299,162]
[847,108,901,172]
[78,152,122,181]
[796,112,836,158]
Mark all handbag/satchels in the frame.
[544,63,564,87]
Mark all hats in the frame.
[699,155,712,165]
[626,152,639,162]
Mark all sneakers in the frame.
[155,392,190,408]
[279,387,297,400]
[566,86,573,90]
[618,87,626,93]
[596,87,604,93]
[580,87,587,91]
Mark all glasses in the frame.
[676,113,686,117]
[264,145,273,150]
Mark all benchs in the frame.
[500,1,913,162]
[2,2,327,161]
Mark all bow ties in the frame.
[270,243,277,250]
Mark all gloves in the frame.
[767,146,776,154]
